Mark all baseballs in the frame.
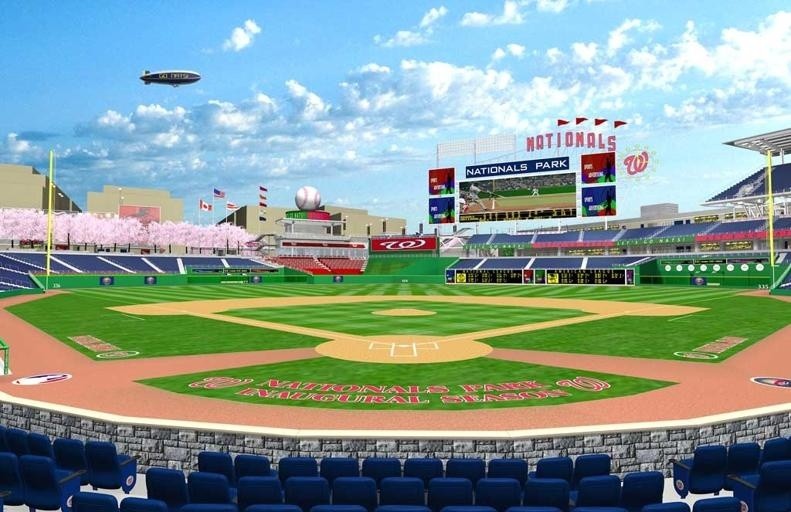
[296,186,321,210]
